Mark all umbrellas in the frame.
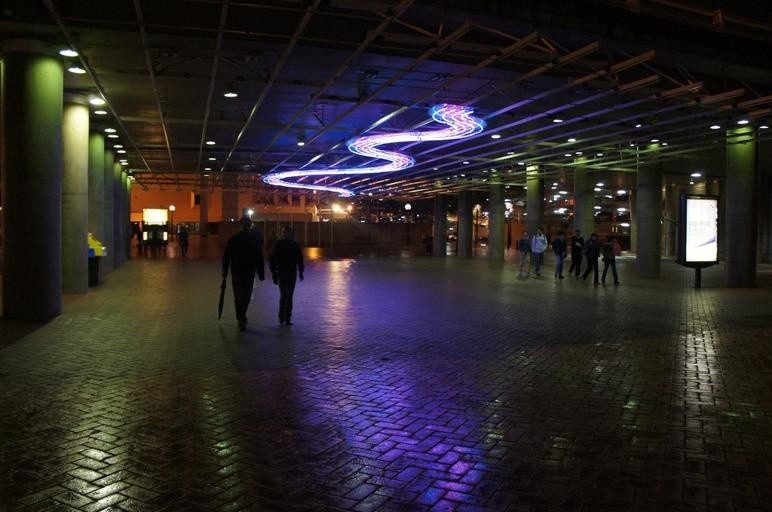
[218,277,226,320]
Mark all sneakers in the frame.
[237,319,294,331]
[516,272,620,286]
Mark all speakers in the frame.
[195,195,201,204]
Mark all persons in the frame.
[250,222,264,245]
[130,219,189,259]
[222,216,264,331]
[518,228,620,288]
[268,226,305,326]
